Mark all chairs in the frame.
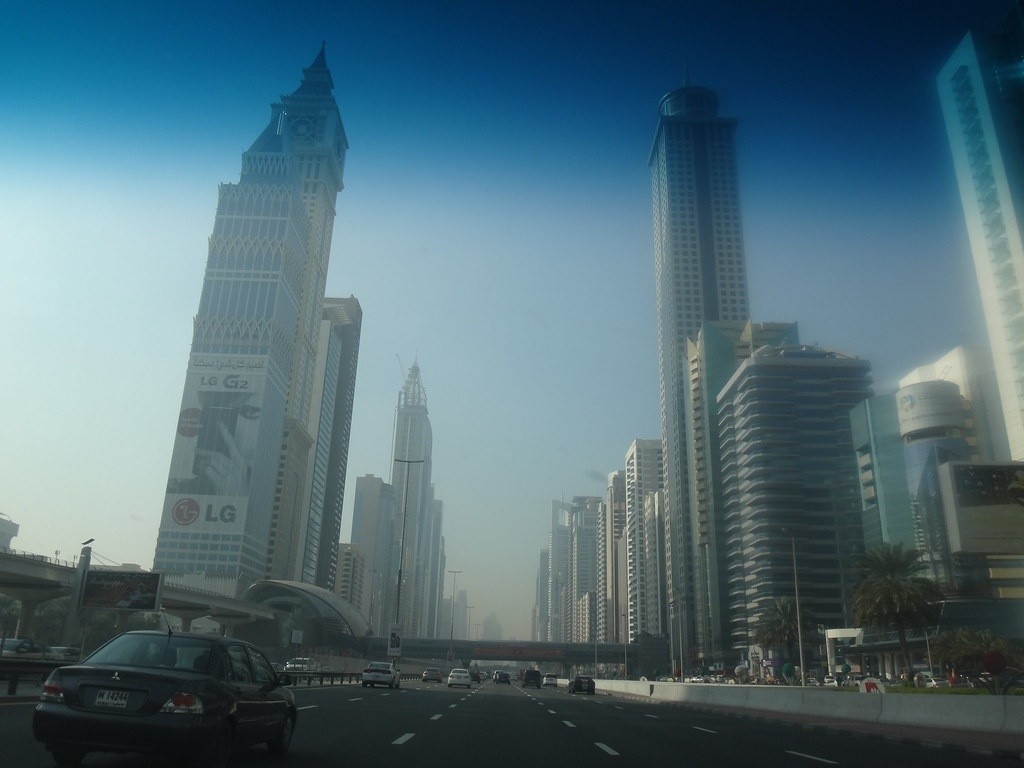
[151,647,176,668]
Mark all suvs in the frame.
[521,669,541,688]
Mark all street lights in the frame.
[446,571,462,662]
[780,527,804,687]
[474,624,481,640]
[622,614,628,678]
[466,606,474,640]
[391,458,425,669]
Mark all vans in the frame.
[543,673,557,688]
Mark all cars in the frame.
[568,674,595,695]
[32,629,298,767]
[447,669,471,688]
[806,674,870,686]
[470,670,517,685]
[659,674,736,684]
[422,667,443,683]
[1,637,81,662]
[286,657,317,670]
[978,671,993,683]
[750,677,769,686]
[925,677,949,689]
[362,662,401,689]
